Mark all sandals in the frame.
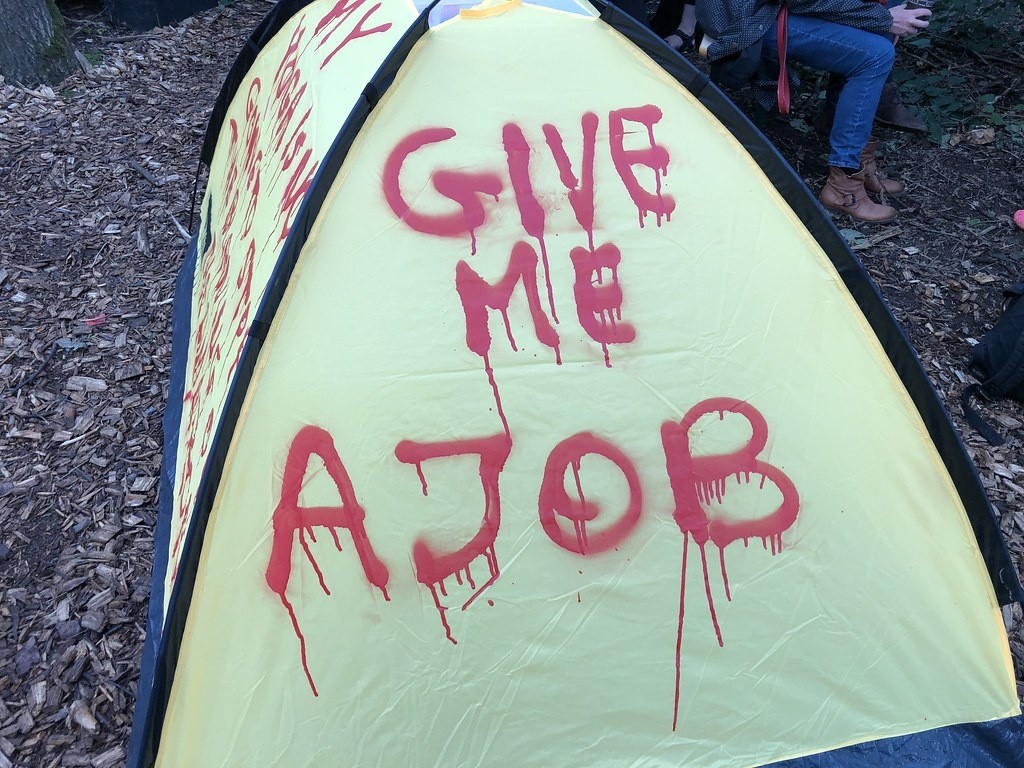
[654,30,695,56]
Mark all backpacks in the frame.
[958,281,1024,446]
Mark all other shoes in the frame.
[1013,210,1024,230]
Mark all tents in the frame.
[124,1,1024,768]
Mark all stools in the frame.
[699,35,783,132]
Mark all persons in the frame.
[653,0,698,52]
[694,1,932,224]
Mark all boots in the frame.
[858,135,905,198]
[819,165,897,223]
[874,82,930,133]
[815,102,835,136]
